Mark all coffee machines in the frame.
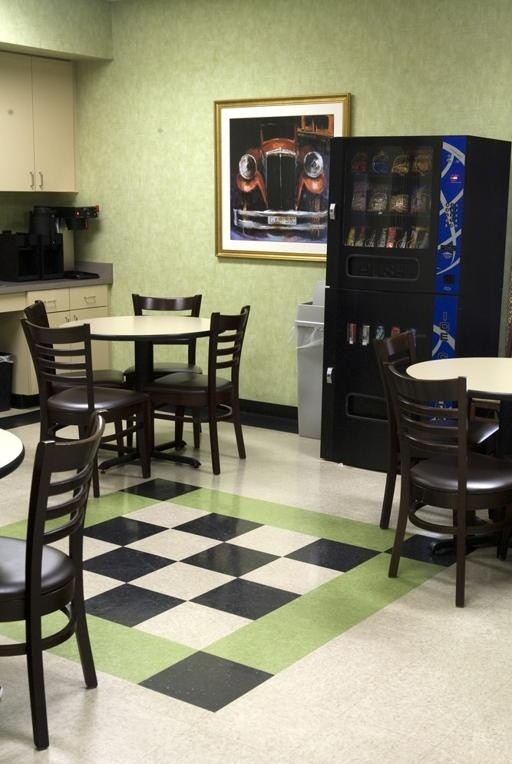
[31,204,100,280]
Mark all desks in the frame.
[406,357,512,561]
[58,316,226,473]
[0,428,25,480]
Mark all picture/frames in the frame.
[213,94,351,261]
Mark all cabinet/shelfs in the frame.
[2,286,108,408]
[0,50,78,193]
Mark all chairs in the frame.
[147,305,250,474]
[19,318,151,497]
[0,409,109,749]
[123,294,203,450]
[371,329,500,529]
[24,300,126,458]
[380,361,512,607]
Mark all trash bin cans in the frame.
[294,302,325,439]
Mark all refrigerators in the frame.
[320,135,512,474]
